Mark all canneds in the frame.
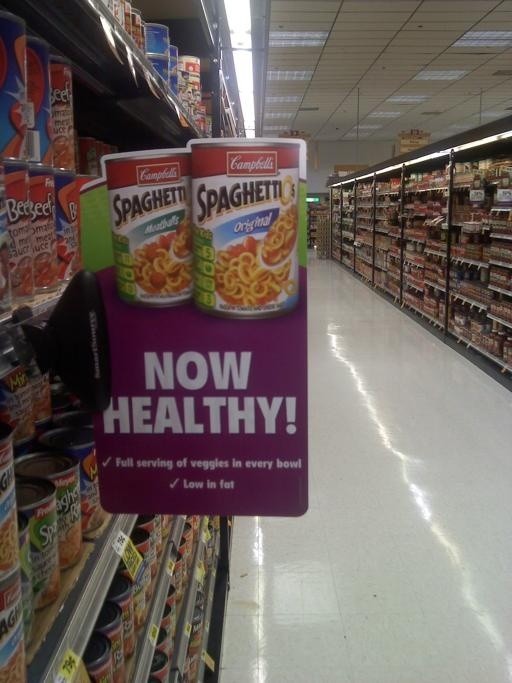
[104,152,193,309]
[0,10,118,314]
[190,141,300,320]
[85,512,175,682]
[1,373,115,682]
[124,0,211,136]
[148,515,203,681]
